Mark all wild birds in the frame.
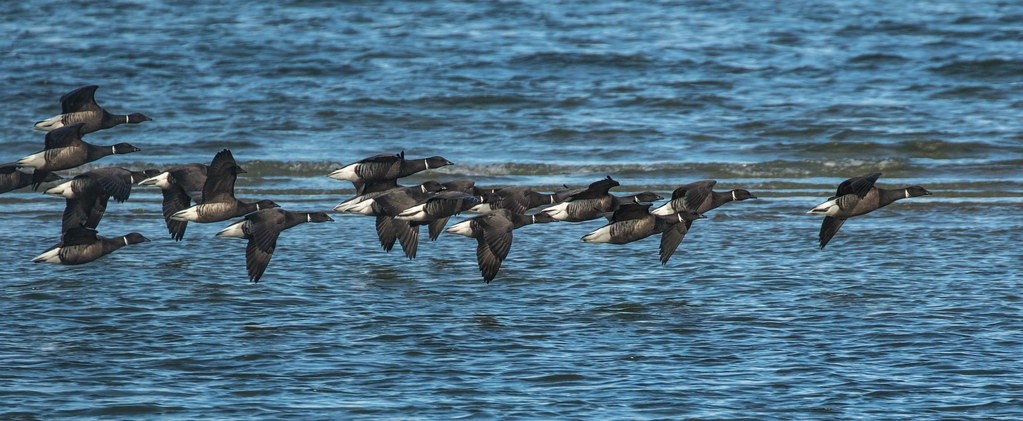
[14,123,140,194]
[214,209,335,284]
[41,166,160,232]
[579,203,706,266]
[324,150,454,200]
[136,162,212,242]
[650,180,758,217]
[0,163,65,193]
[30,224,150,265]
[168,149,281,223]
[326,176,621,285]
[806,173,933,251]
[34,85,152,134]
[541,191,665,225]
